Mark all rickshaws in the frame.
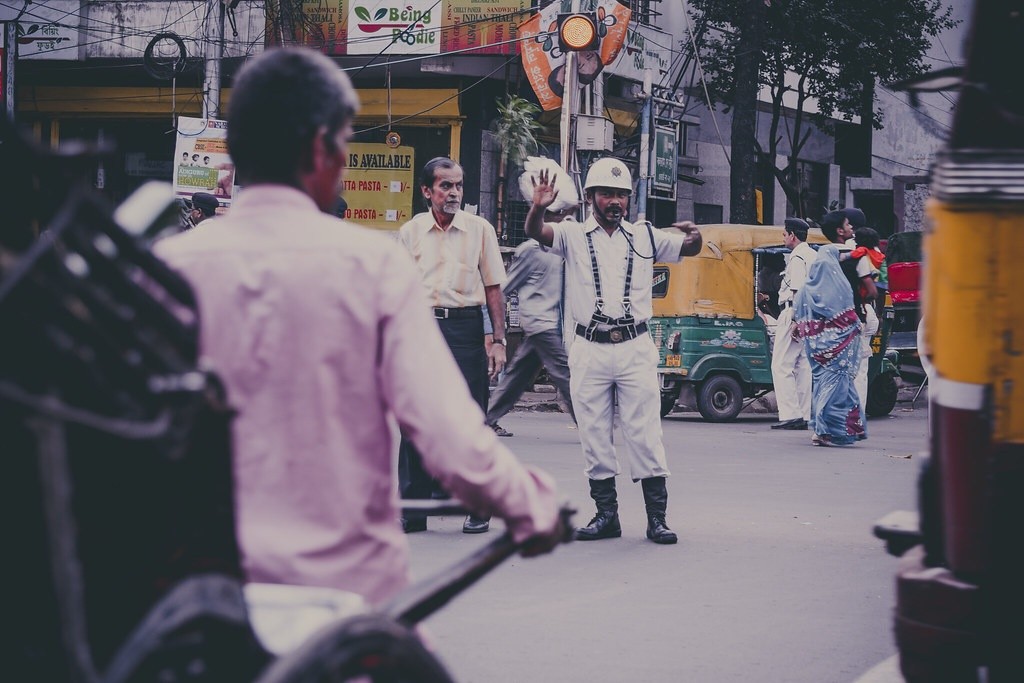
[0,118,587,683]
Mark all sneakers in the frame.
[575,511,622,539]
[646,512,678,543]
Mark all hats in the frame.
[188,192,219,211]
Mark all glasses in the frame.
[189,208,198,214]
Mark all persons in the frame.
[486,219,578,439]
[394,156,510,535]
[180,152,210,168]
[524,158,702,545]
[150,50,571,607]
[190,191,218,225]
[0,115,279,683]
[770,217,818,430]
[811,207,881,447]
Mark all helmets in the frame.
[582,158,632,196]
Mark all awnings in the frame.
[13,87,459,127]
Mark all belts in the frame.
[778,301,792,309]
[433,308,482,319]
[575,322,647,343]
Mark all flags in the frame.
[514,0,638,112]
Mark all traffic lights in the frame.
[557,12,598,52]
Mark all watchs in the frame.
[493,338,507,346]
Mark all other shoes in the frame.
[492,425,513,436]
[771,417,808,430]
[462,514,489,532]
[401,514,427,533]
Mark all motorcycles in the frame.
[647,223,901,423]
